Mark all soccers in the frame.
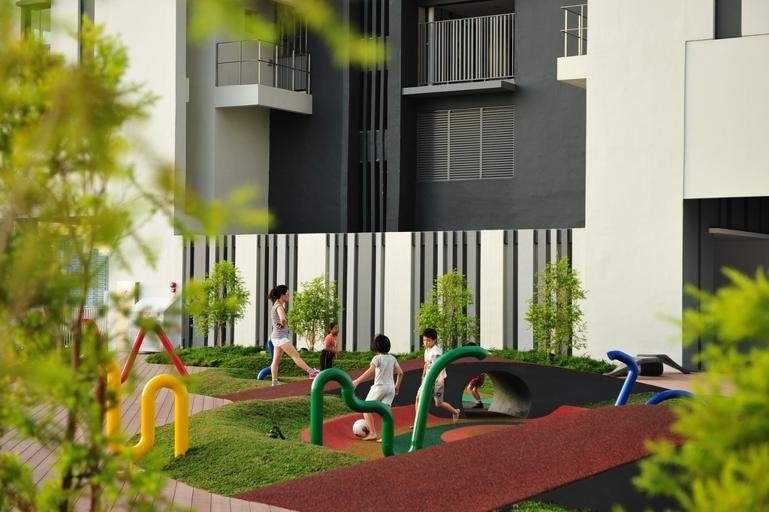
[353,419,370,437]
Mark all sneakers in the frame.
[272,380,281,386]
[472,403,484,408]
[309,368,320,379]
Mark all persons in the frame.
[320,322,339,370]
[409,327,460,430]
[467,341,485,407]
[268,286,320,386]
[352,333,404,442]
